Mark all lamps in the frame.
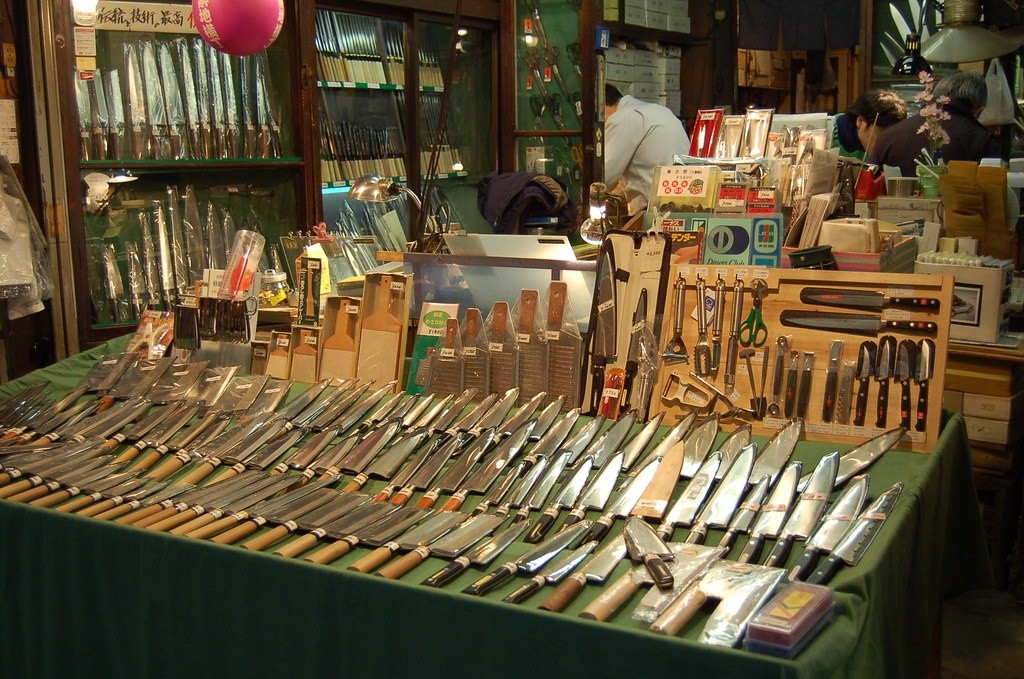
[892,0,944,77]
[347,172,445,254]
[94,168,139,214]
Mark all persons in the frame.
[604,83,695,215]
[870,69,1003,176]
[826,89,909,164]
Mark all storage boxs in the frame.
[603,47,634,65]
[658,91,681,116]
[645,10,667,30]
[659,74,679,91]
[780,236,918,274]
[631,82,660,98]
[914,261,1014,344]
[659,48,681,58]
[604,63,635,83]
[633,66,658,83]
[605,80,632,96]
[625,0,644,9]
[667,0,688,17]
[658,58,680,75]
[644,0,667,14]
[633,51,659,67]
[666,14,690,33]
[625,6,645,26]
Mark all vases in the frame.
[917,164,948,200]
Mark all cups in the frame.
[788,244,838,270]
[916,165,947,199]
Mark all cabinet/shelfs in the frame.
[0,0,598,386]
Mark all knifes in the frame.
[75,8,489,181]
[0,191,945,650]
[697,108,829,188]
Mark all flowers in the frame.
[916,71,951,163]
